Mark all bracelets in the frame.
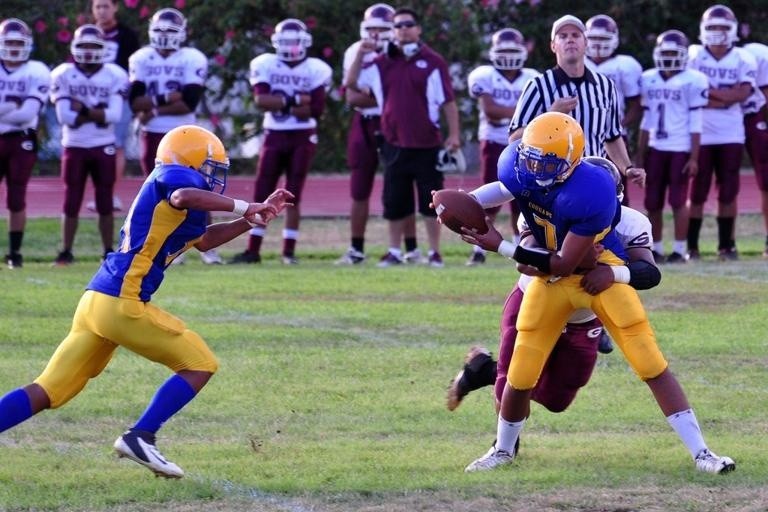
[242,213,263,228]
[282,93,301,107]
[231,198,250,217]
[78,103,89,117]
[151,92,171,107]
[280,105,292,115]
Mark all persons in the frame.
[343,4,461,267]
[0,124,295,479]
[0,17,50,267]
[85,0,139,212]
[49,24,126,267]
[508,14,646,355]
[429,112,735,475]
[126,8,223,267]
[466,5,768,267]
[225,17,334,266]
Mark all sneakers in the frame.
[690,446,739,477]
[378,249,486,268]
[112,428,185,482]
[5,253,24,270]
[445,346,494,412]
[464,441,516,480]
[650,242,738,265]
[224,249,300,266]
[52,251,75,268]
[201,248,225,266]
[332,248,368,270]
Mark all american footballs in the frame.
[433,189,489,235]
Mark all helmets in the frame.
[70,24,108,65]
[512,111,587,194]
[146,7,188,51]
[652,28,689,72]
[585,13,619,58]
[584,156,627,205]
[153,124,231,197]
[489,27,528,72]
[698,3,740,47]
[360,3,396,46]
[0,17,33,62]
[270,18,312,64]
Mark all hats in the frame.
[550,14,587,43]
